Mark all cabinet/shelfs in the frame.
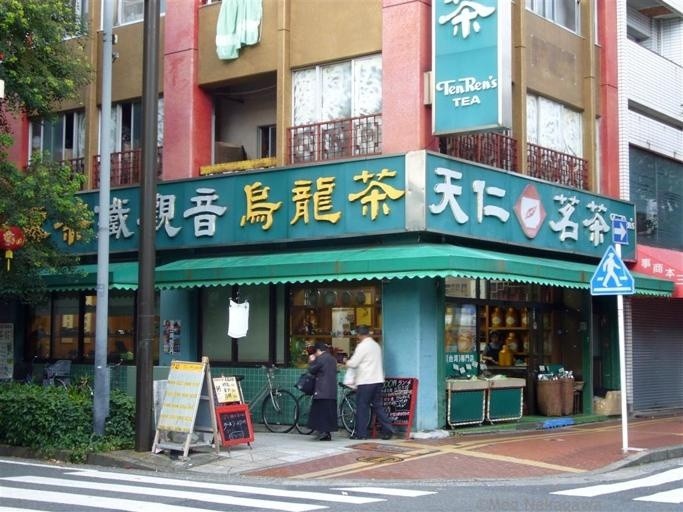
[443,304,563,368]
[289,283,381,372]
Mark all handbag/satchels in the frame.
[294,372,315,396]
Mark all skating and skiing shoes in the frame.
[315,431,331,441]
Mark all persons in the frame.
[306,343,338,441]
[482,330,504,365]
[343,325,396,440]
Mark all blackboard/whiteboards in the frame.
[216,404,254,446]
[382,378,417,425]
[157,361,204,433]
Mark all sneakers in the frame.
[381,431,393,440]
[350,435,367,440]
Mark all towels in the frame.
[225,298,249,339]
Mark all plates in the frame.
[309,289,366,306]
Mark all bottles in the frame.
[498,345,512,367]
[491,307,529,353]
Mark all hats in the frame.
[489,331,500,337]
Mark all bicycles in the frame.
[21,353,91,396]
[293,368,377,435]
[233,362,299,434]
